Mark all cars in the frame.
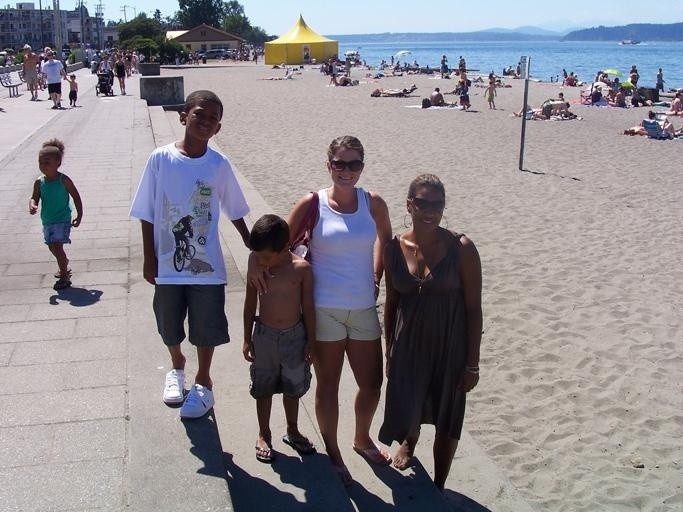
[226,48,237,57]
[51,47,71,61]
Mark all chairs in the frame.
[641,118,671,141]
[580,83,594,105]
[378,84,417,97]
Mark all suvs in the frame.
[197,48,225,59]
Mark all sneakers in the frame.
[178,385,214,421]
[30,95,76,109]
[163,368,188,405]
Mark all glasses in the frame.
[407,196,446,214]
[328,159,364,173]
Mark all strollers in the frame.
[94,69,114,96]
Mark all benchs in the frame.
[17,70,41,91]
[0,73,22,98]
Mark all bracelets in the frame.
[375,284,380,289]
[466,365,480,375]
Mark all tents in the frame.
[264,12,338,64]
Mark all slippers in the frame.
[336,463,352,485]
[282,434,316,454]
[53,280,72,291]
[352,442,392,467]
[254,439,276,462]
[54,266,72,277]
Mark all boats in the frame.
[615,37,650,47]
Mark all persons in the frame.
[29,141,82,290]
[130,92,252,416]
[513,92,578,121]
[640,110,682,139]
[242,213,315,461]
[283,51,372,86]
[246,135,393,485]
[170,43,264,65]
[555,65,682,115]
[3,35,144,107]
[370,55,522,111]
[380,172,481,496]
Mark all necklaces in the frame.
[412,233,435,293]
